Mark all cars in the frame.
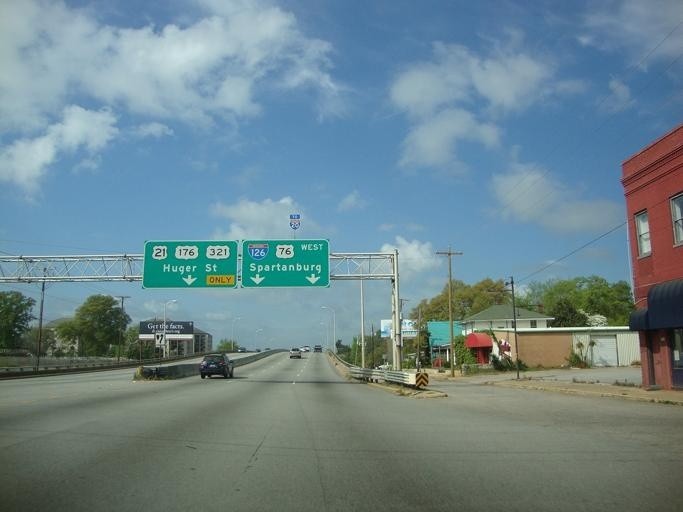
[264,348,270,352]
[256,348,261,352]
[237,347,247,353]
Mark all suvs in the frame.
[199,353,234,379]
[288,343,322,358]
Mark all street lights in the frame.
[231,318,240,350]
[318,305,336,353]
[254,329,263,348]
[115,295,130,364]
[505,277,520,379]
[162,299,177,358]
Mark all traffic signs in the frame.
[141,240,238,289]
[240,239,331,289]
[288,214,301,231]
